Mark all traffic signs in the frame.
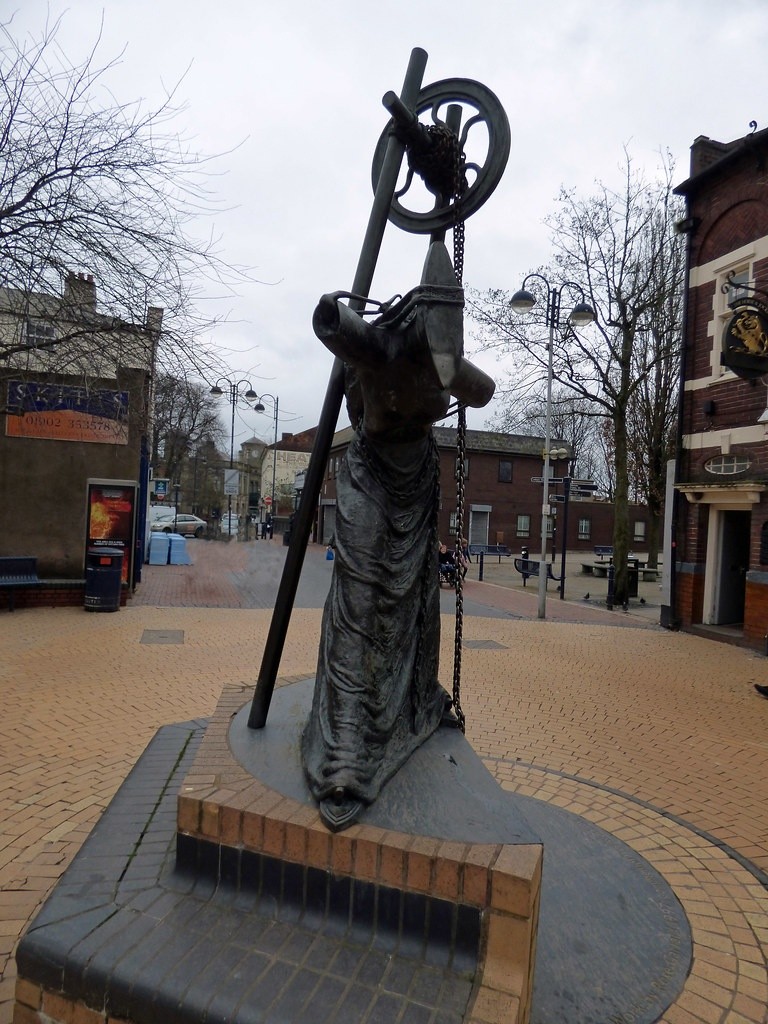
[530,476,597,503]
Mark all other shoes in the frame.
[446,578,451,583]
[462,579,465,583]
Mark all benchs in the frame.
[0,556,39,612]
[594,545,613,560]
[514,558,567,591]
[469,544,511,564]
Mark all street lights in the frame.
[209,377,259,536]
[254,393,279,539]
[509,273,597,618]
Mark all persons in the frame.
[328,533,336,549]
[312,242,497,831]
[439,537,472,589]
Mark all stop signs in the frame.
[264,496,272,506]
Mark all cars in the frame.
[150,513,208,539]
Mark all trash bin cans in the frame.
[84,547,125,612]
[283,530,291,546]
[609,558,639,597]
